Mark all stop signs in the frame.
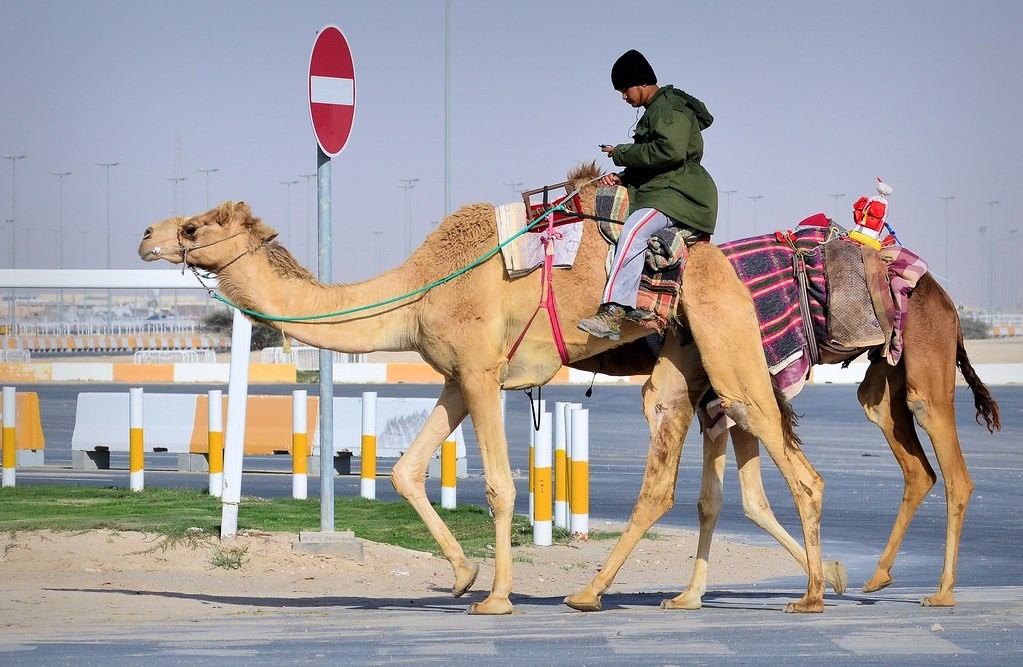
[305,23,358,160]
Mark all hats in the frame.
[611,49,657,91]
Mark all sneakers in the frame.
[577,303,626,341]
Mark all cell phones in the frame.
[601,144,607,148]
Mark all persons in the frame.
[578,49,718,340]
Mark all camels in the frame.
[138,161,1003,615]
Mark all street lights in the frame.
[196,168,218,316]
[1,155,26,337]
[829,193,846,224]
[983,199,1000,317]
[48,170,70,321]
[165,176,186,332]
[503,181,522,204]
[277,180,297,251]
[393,177,420,263]
[719,189,736,244]
[295,173,318,270]
[96,162,119,333]
[746,195,763,237]
[937,195,955,294]
